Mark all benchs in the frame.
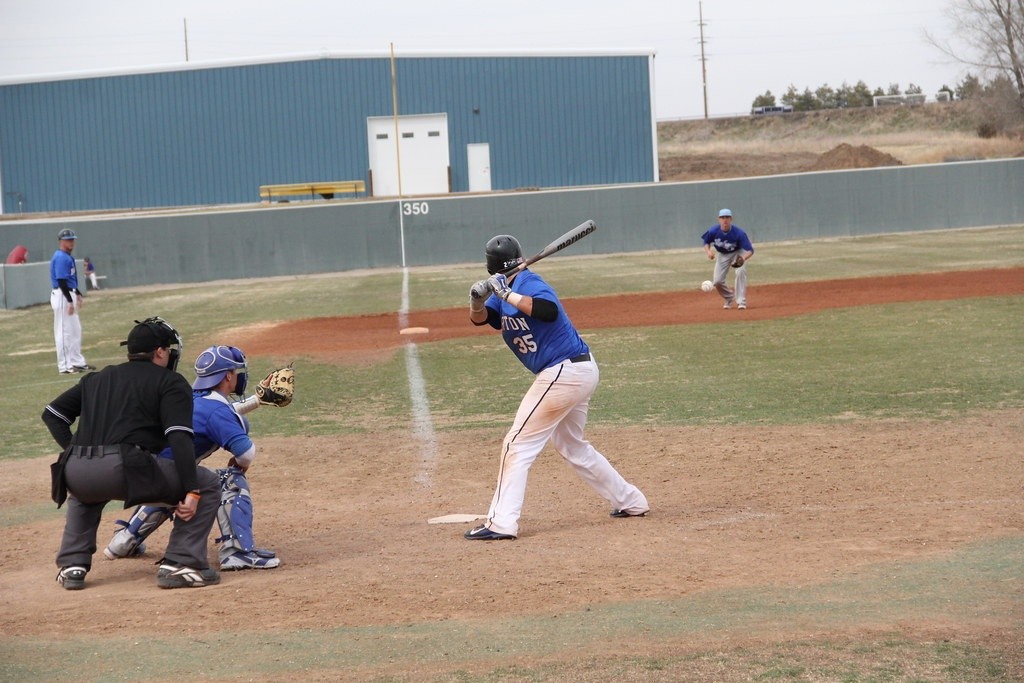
[96,276,107,279]
[260,181,365,203]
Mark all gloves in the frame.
[470,278,493,313]
[486,274,513,302]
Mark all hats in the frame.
[127,319,179,355]
[718,210,732,217]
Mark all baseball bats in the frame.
[471,218,597,298]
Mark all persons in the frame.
[84,257,99,290]
[41,314,222,587]
[103,344,281,572]
[701,208,755,310]
[463,235,650,540]
[50,229,97,374]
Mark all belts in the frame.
[71,444,122,456]
[570,352,590,364]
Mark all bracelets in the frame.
[188,492,200,499]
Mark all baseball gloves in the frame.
[732,253,744,268]
[254,360,295,408]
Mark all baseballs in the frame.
[701,280,713,292]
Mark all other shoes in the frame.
[723,298,733,309]
[738,304,746,310]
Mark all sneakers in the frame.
[464,523,515,540]
[73,363,96,372]
[60,369,77,376]
[610,505,647,517]
[157,557,219,589]
[220,549,280,572]
[105,541,147,560]
[58,564,88,589]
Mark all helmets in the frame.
[57,228,77,240]
[190,345,236,390]
[485,235,525,275]
